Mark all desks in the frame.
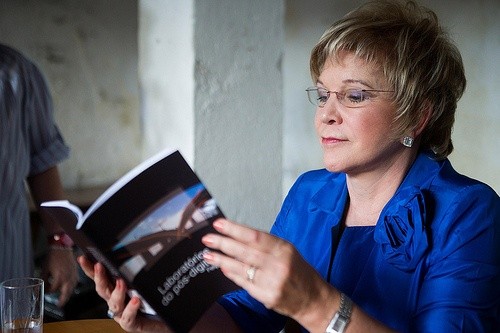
[43,319,172,333]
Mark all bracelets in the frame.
[46,232,73,246]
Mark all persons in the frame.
[0,44,79,333]
[77,0,500,333]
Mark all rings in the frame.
[248,266,257,280]
[106,309,116,319]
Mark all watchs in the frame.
[325,293,353,333]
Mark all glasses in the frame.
[304,86,397,108]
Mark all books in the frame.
[40,149,243,333]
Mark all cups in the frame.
[0,278,44,333]
